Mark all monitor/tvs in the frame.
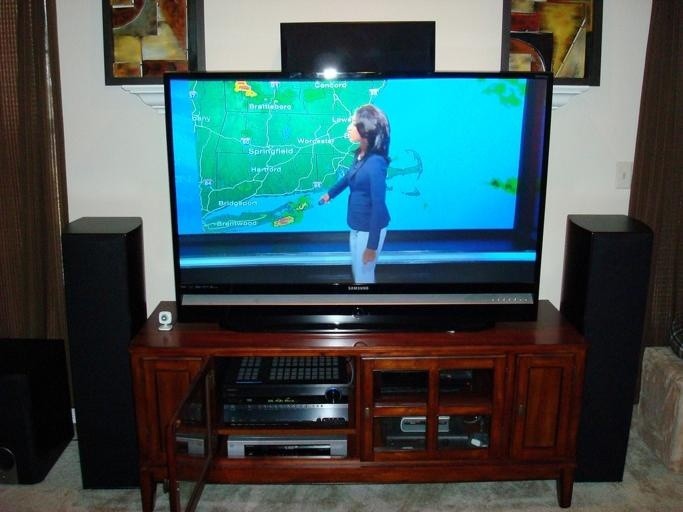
[164,69,555,333]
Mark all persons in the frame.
[322,103,393,284]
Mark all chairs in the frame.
[640,346,683,474]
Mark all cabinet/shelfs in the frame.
[128,300,590,512]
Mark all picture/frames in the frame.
[102,1,197,89]
[501,0,603,88]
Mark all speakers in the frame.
[561,213,654,483]
[61,216,148,489]
